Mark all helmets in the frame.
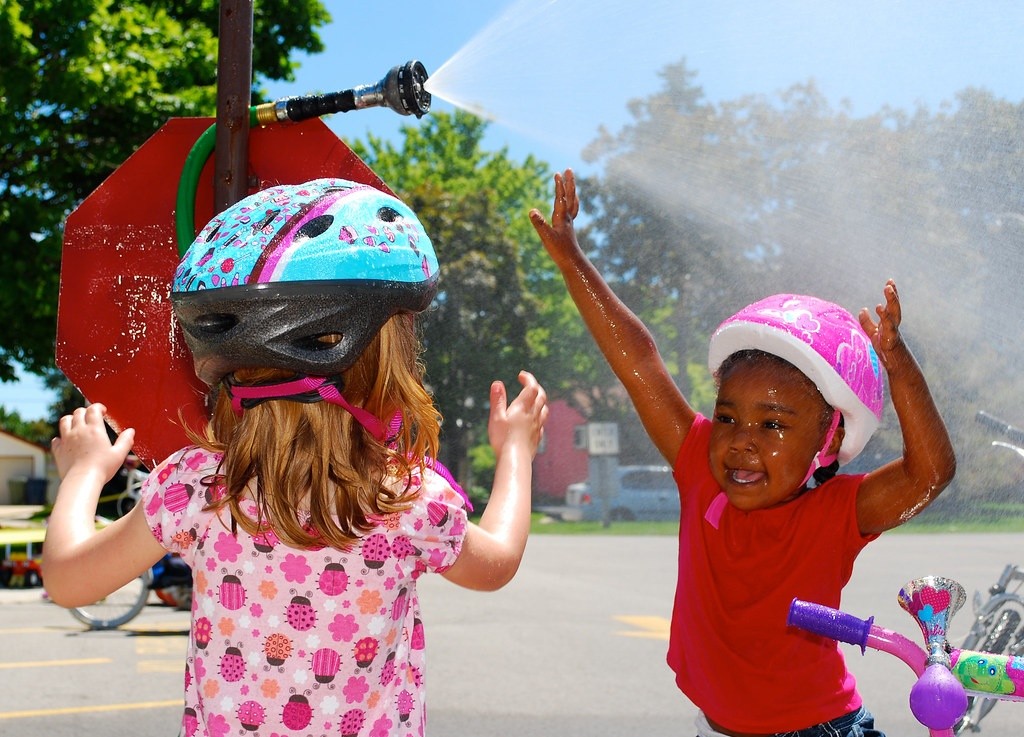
[170,177,443,374]
[707,293,883,467]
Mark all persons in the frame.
[43,179,550,737]
[531,167,956,737]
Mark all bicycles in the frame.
[56,455,196,629]
[787,407,1024,737]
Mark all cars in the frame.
[578,465,681,522]
